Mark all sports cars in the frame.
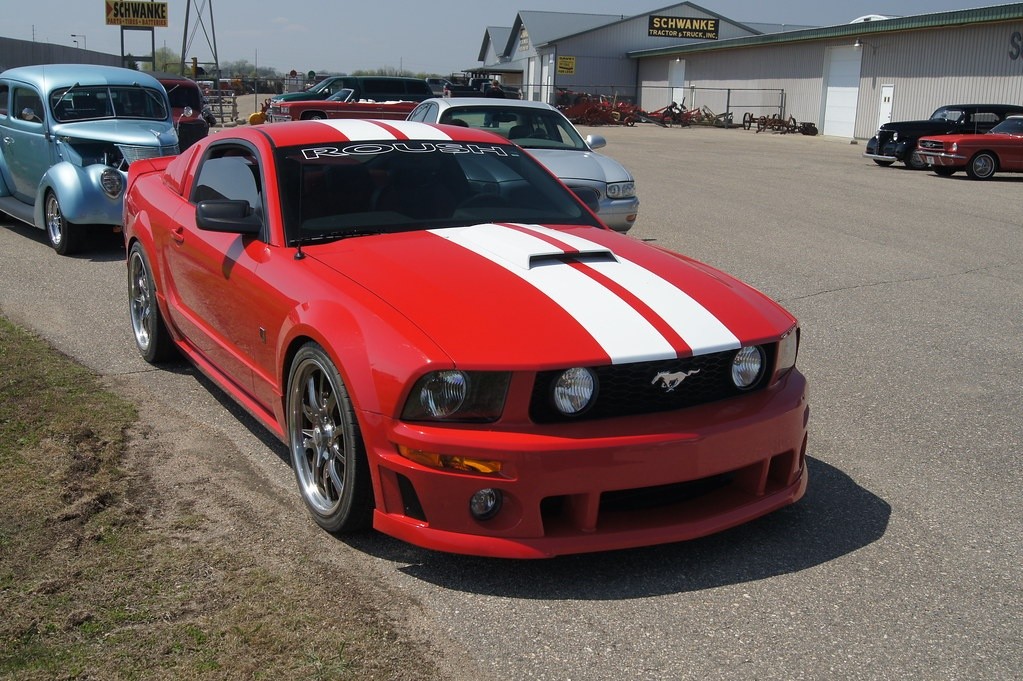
[266,87,418,124]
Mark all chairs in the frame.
[509,126,532,140]
[964,114,972,123]
[450,119,469,128]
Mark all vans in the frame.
[425,77,453,90]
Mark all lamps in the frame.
[676,56,692,65]
[854,38,876,54]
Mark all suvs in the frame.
[862,103,1021,170]
[271,74,436,107]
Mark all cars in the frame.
[406,98,640,232]
[921,116,1023,181]
[0,62,194,258]
[123,118,812,554]
[115,70,217,152]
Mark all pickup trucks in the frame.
[443,78,521,96]
[443,83,519,99]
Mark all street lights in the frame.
[71,34,88,49]
[73,41,78,48]
